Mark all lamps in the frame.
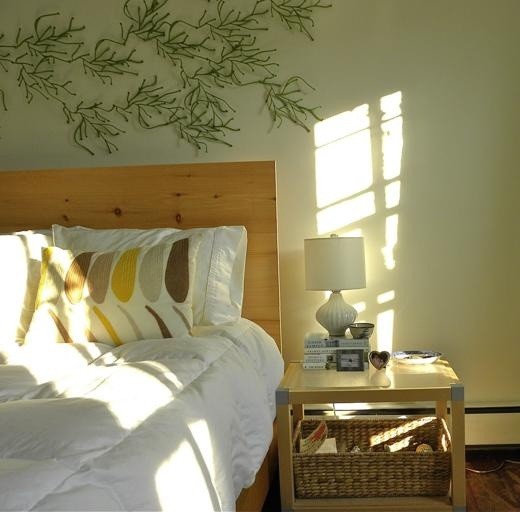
[303,228,369,335]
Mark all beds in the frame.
[2,159,281,508]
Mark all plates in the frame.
[393,350,444,363]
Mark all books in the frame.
[305,354,368,364]
[303,334,369,347]
[298,420,336,453]
[302,363,371,372]
[303,345,370,354]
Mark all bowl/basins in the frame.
[348,322,375,339]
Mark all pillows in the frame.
[51,222,249,328]
[0,226,59,363]
[20,233,204,350]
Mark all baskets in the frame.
[289,414,453,500]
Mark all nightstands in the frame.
[277,354,469,510]
